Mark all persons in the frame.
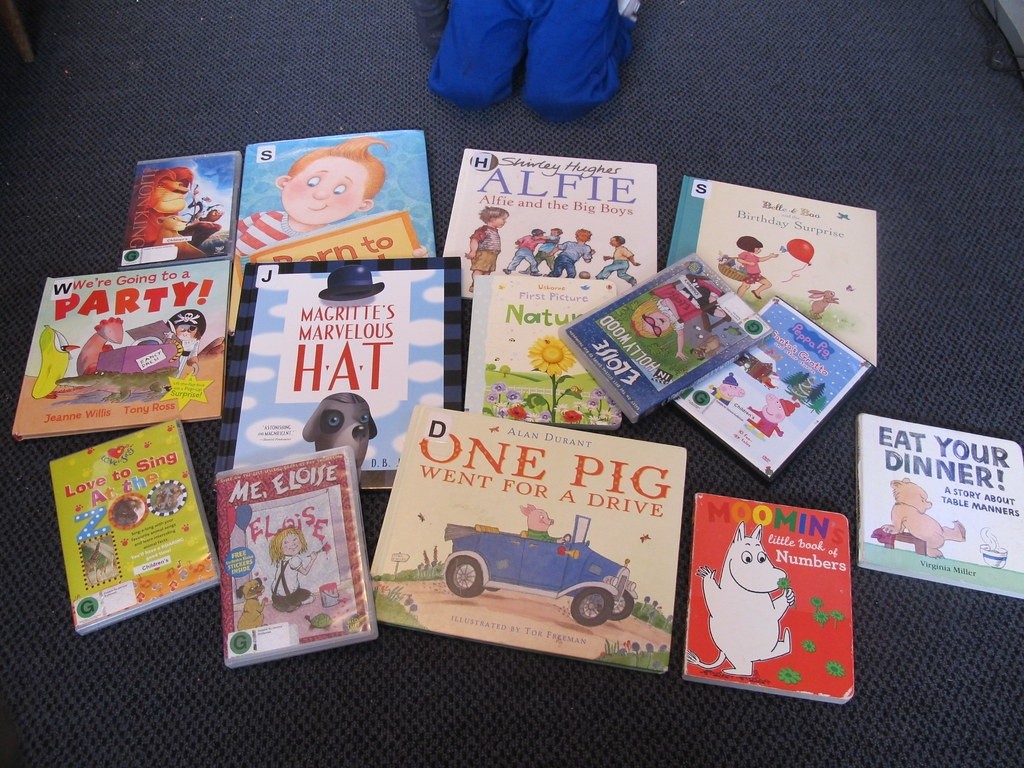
[410,0,637,122]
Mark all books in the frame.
[227,128,435,334]
[674,298,876,481]
[218,444,380,670]
[853,413,1024,599]
[442,148,659,301]
[11,261,230,442]
[118,152,242,271]
[559,252,774,424]
[666,174,879,366]
[48,418,222,636]
[682,493,855,705]
[463,276,623,430]
[369,404,689,676]
[214,256,464,490]
